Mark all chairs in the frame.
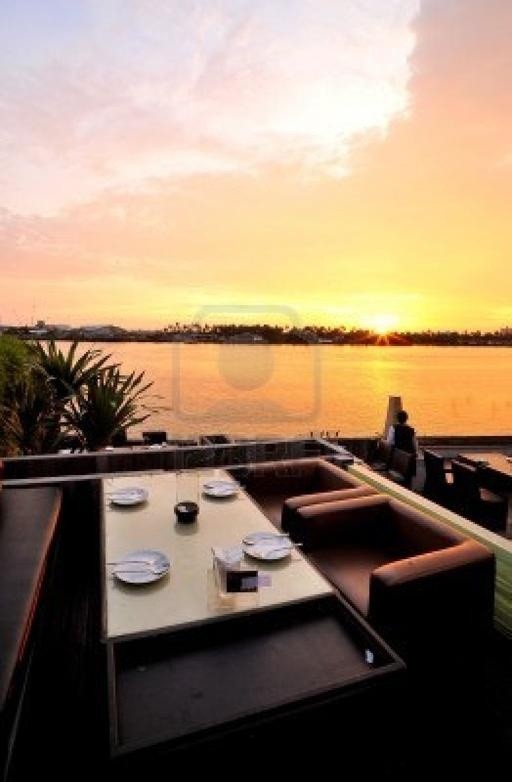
[423,449,512,528]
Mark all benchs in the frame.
[1,486,66,780]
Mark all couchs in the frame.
[241,457,379,533]
[289,493,498,632]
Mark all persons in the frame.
[387,410,419,490]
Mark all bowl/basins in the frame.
[175,502,200,523]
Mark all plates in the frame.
[242,531,291,561]
[112,486,147,506]
[114,549,170,584]
[203,480,239,498]
